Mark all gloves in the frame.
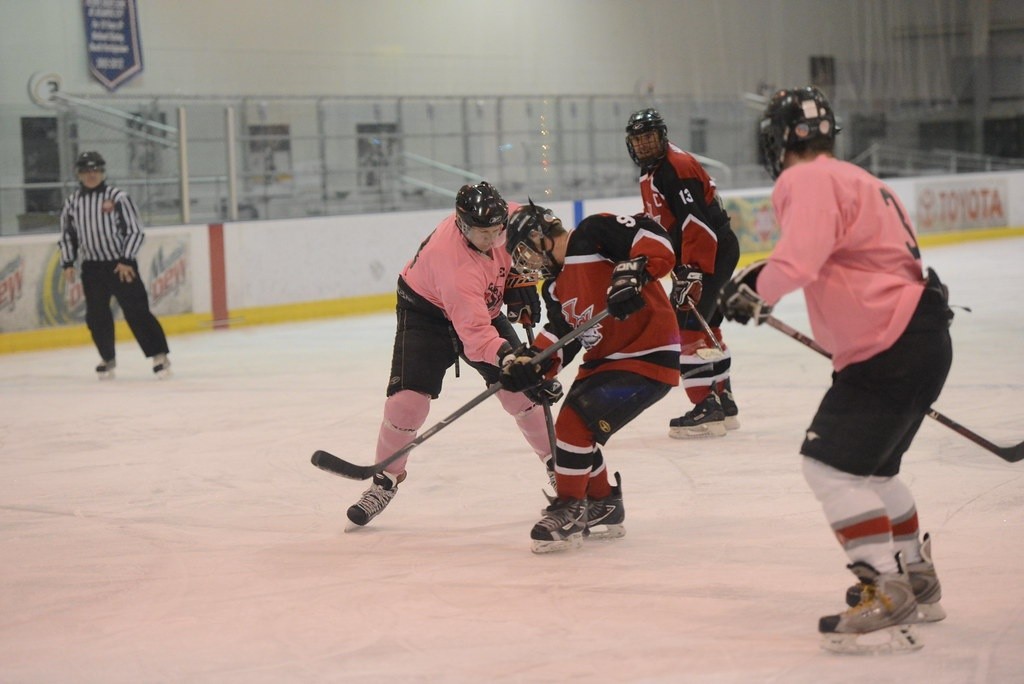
[670,262,702,312]
[720,280,772,326]
[524,378,564,406]
[503,269,540,324]
[499,343,541,392]
[606,257,649,321]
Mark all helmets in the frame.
[455,181,508,227]
[505,205,562,279]
[625,108,667,167]
[757,85,840,180]
[75,152,105,171]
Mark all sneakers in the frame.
[669,380,727,439]
[530,481,591,553]
[718,377,739,430]
[152,352,171,377]
[819,549,925,654]
[845,533,946,623]
[586,472,626,540]
[344,470,407,534]
[544,452,557,493]
[96,356,116,378]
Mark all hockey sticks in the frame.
[669,269,725,361]
[763,311,1024,463]
[518,310,558,465]
[310,308,610,479]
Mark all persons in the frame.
[58,150,172,380]
[504,204,681,553]
[345,181,563,532]
[717,88,953,654]
[625,108,740,440]
